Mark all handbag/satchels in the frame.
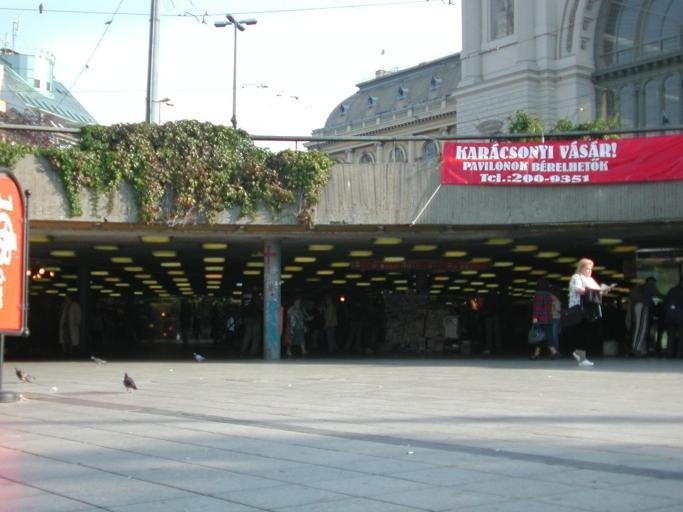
[528,322,545,343]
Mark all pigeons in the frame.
[123,371,139,394]
[92,353,107,365]
[193,349,207,363]
[38,2,43,12]
[15,369,26,385]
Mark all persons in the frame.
[547,292,564,359]
[57,292,83,360]
[568,254,612,367]
[630,274,668,359]
[280,296,340,357]
[225,312,259,356]
[527,279,551,361]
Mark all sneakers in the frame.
[572,352,581,361]
[579,359,594,367]
